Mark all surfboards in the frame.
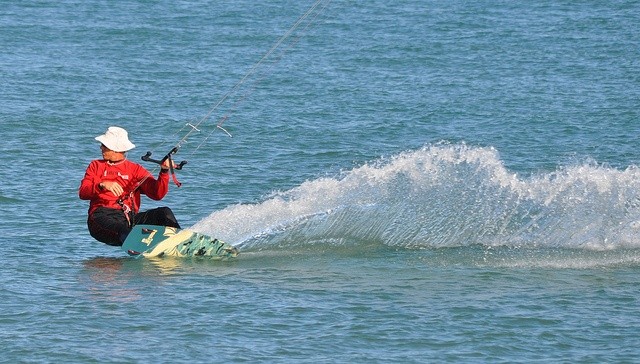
[120,224,240,263]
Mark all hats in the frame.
[95,126,136,152]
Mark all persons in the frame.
[79,126,181,246]
